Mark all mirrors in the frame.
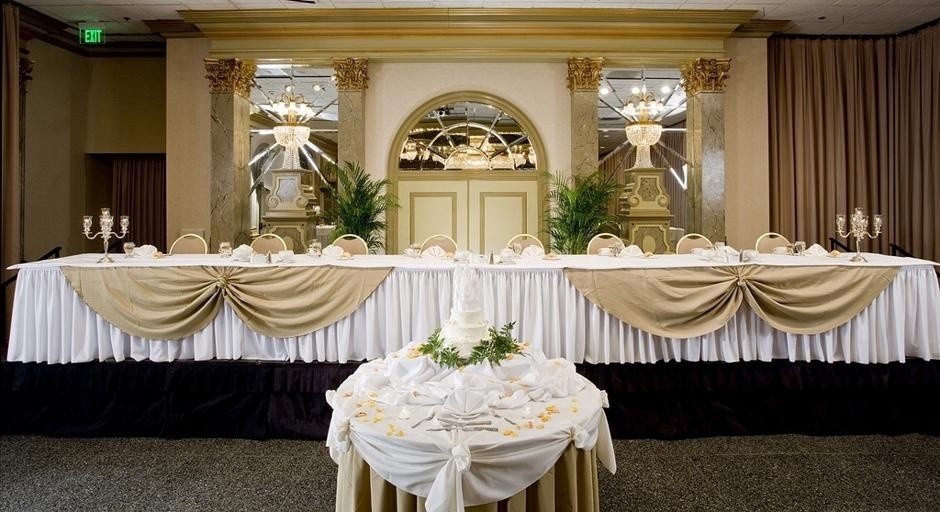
[245,63,340,249]
[595,60,693,251]
[395,93,540,174]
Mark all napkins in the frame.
[420,245,447,257]
[806,243,829,254]
[321,245,344,254]
[618,245,644,256]
[134,244,159,259]
[718,245,739,254]
[231,243,259,258]
[522,244,544,255]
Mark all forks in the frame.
[407,410,516,434]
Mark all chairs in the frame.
[169,232,207,256]
[675,233,715,254]
[330,234,369,255]
[251,233,287,253]
[586,233,626,253]
[419,234,459,253]
[755,232,791,253]
[506,232,545,255]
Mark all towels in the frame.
[358,337,588,432]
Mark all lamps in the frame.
[267,85,316,170]
[622,83,663,170]
[835,208,883,263]
[79,206,132,264]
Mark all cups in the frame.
[120,238,808,264]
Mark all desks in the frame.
[1,253,940,365]
